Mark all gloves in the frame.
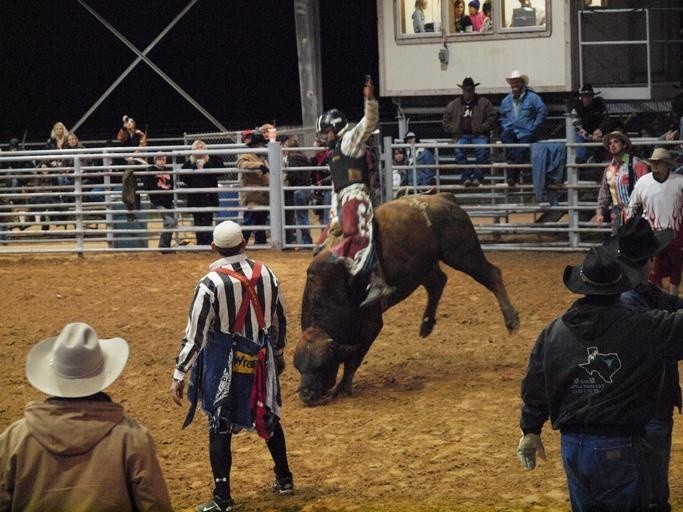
[516,430,546,471]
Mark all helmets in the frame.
[314,109,349,149]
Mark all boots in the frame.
[361,261,388,308]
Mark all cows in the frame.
[293,191,521,409]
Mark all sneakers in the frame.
[195,494,232,512]
[272,472,293,494]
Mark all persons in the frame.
[411,0,427,34]
[453,1,472,32]
[506,0,545,27]
[480,0,492,32]
[143,151,177,253]
[0,319,173,511]
[517,244,683,511]
[467,1,483,32]
[4,122,93,222]
[608,217,683,511]
[118,117,146,165]
[393,132,436,194]
[575,84,683,237]
[235,124,332,247]
[309,74,395,311]
[443,70,548,190]
[179,140,224,247]
[168,220,293,512]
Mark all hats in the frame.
[213,220,243,249]
[26,323,129,399]
[468,0,480,9]
